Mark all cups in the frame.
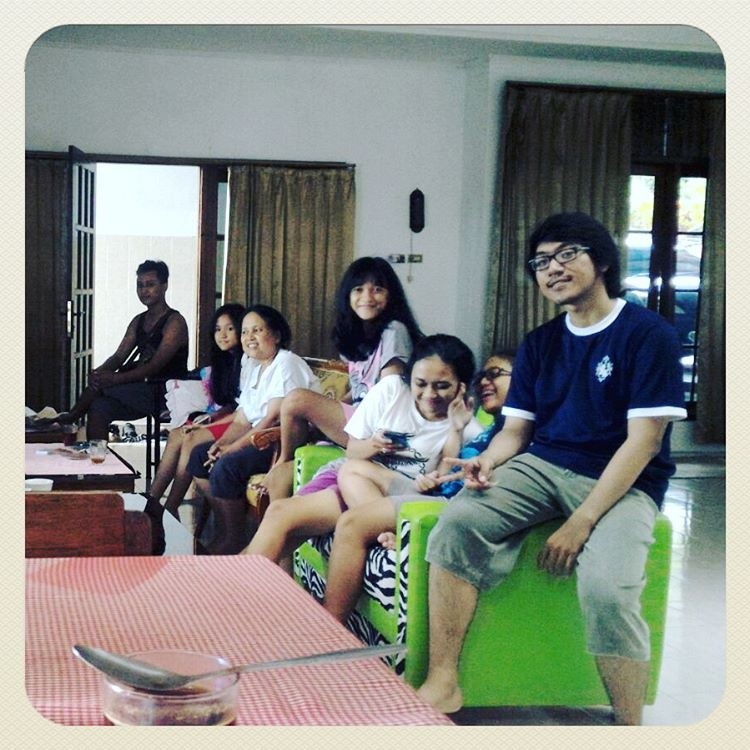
[102,649,241,726]
[64,424,79,445]
[89,439,108,464]
[25,479,53,493]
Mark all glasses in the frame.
[525,246,591,274]
[471,367,512,387]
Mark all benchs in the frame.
[165,356,350,538]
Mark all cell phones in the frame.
[383,431,408,452]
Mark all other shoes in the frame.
[122,423,137,437]
[108,424,122,442]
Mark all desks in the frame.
[25,555,458,727]
[25,422,153,556]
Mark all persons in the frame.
[55,260,189,445]
[415,214,687,725]
[151,257,512,624]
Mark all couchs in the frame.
[292,408,672,705]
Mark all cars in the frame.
[622,270,702,346]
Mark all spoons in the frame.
[71,643,408,692]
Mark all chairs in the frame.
[146,371,196,494]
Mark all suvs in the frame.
[626,235,703,276]
